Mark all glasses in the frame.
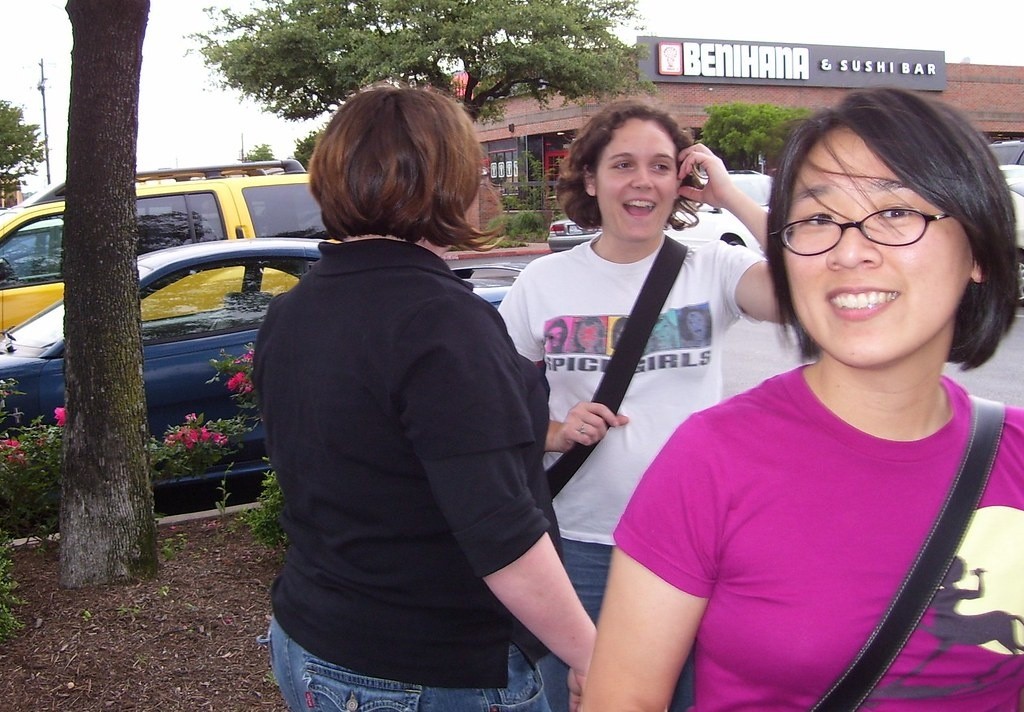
[770,209,953,256]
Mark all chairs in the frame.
[209,292,245,332]
[240,293,274,327]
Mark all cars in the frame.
[668,167,776,253]
[1,237,331,528]
[547,215,604,253]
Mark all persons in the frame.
[498,99,789,712]
[583,88,1024,712]
[252,89,597,712]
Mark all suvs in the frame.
[0,161,343,339]
[988,140,1024,307]
